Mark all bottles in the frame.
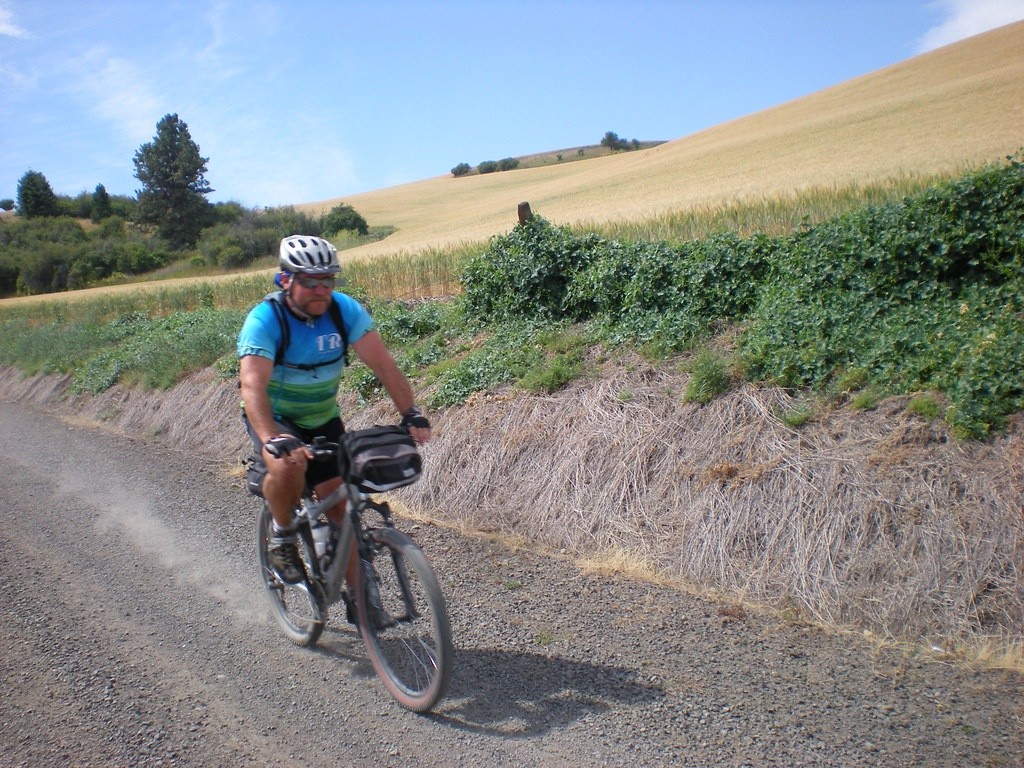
[311,519,330,558]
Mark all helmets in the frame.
[280,235,342,274]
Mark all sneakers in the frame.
[346,601,395,627]
[269,521,305,583]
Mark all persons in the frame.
[235,234,431,626]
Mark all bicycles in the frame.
[255,425,453,711]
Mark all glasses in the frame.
[284,270,337,288]
